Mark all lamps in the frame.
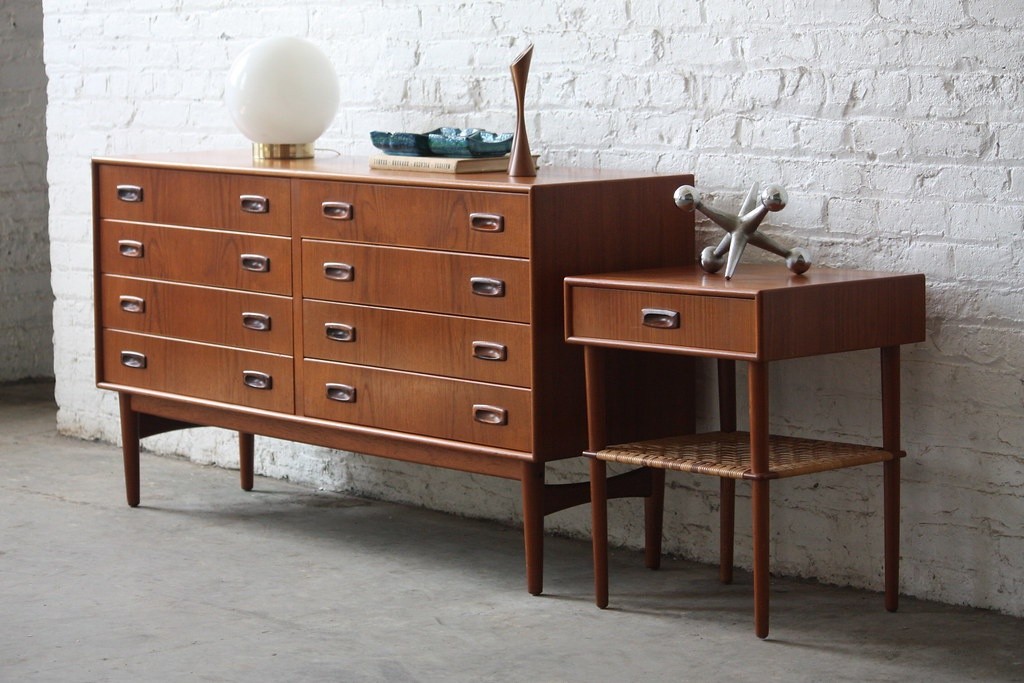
[227,36,340,159]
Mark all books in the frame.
[370,153,540,172]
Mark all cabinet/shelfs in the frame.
[564,263,927,639]
[91,150,696,597]
